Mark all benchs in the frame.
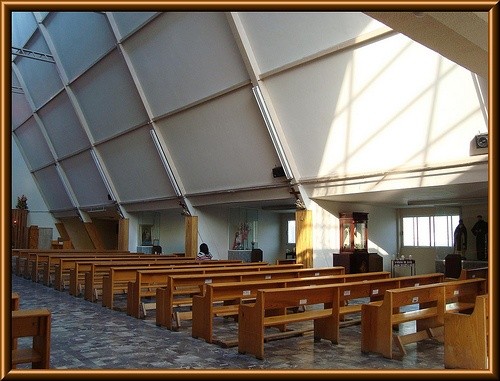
[10,248,490,371]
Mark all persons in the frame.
[195,243,213,261]
[471,219,488,260]
[454,220,467,251]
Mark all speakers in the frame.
[108,194,112,200]
[272,167,285,177]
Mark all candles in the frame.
[252,220,256,244]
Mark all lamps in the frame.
[475,135,488,150]
